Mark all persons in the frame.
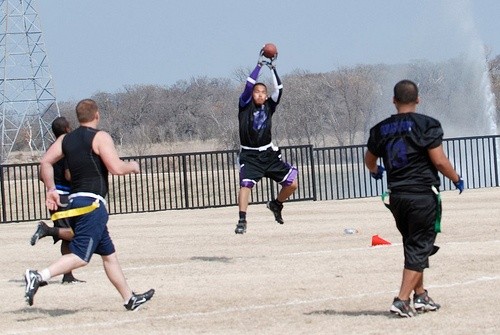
[235,47,298,233]
[366,80,464,317]
[31,116,88,284]
[24,98,156,312]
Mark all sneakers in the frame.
[123,289,154,310]
[390,289,441,318]
[24,269,48,306]
[235,219,247,234]
[31,221,49,246]
[266,200,284,224]
[62,278,87,285]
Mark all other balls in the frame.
[261,41,278,59]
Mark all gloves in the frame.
[257,47,278,68]
[453,177,464,194]
[371,166,386,180]
